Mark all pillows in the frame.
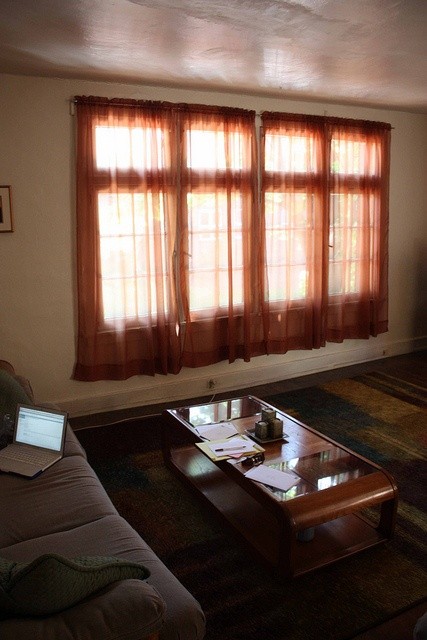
[1,556,151,618]
[0,369,36,447]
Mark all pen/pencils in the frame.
[214,446,246,451]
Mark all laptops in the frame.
[0,401,70,481]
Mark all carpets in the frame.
[68,360,427,640]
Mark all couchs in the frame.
[0,360,208,639]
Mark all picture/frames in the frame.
[0,185,15,232]
